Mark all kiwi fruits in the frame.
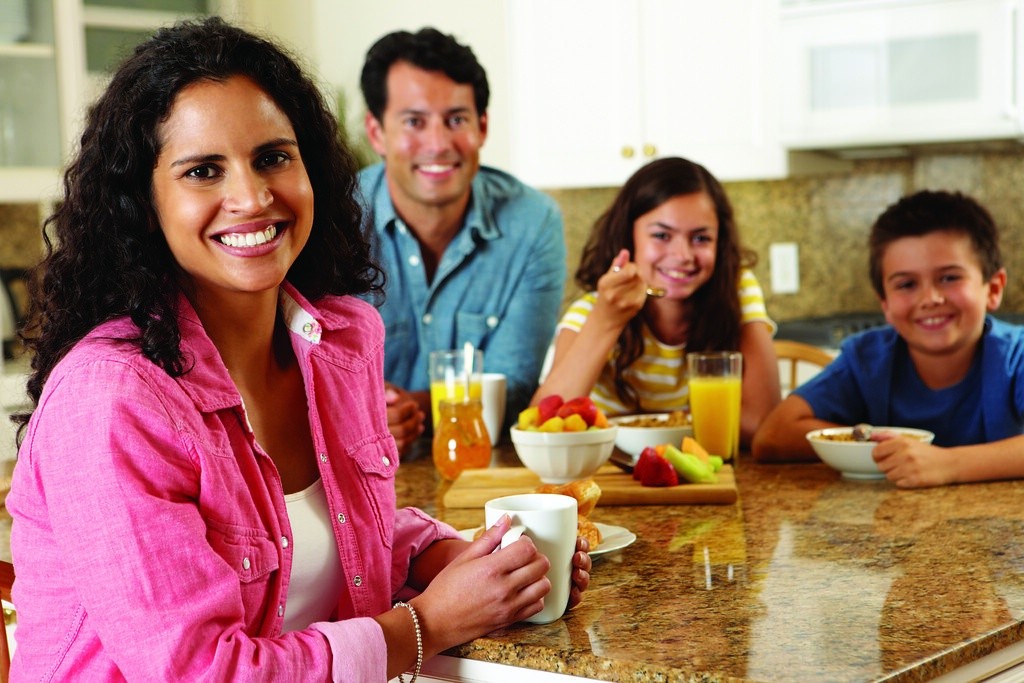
[664,444,723,484]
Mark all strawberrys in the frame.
[538,394,597,427]
[632,447,678,486]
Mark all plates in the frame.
[457,522,637,563]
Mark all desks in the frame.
[396,447,1024,683]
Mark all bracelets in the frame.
[392,601,423,683]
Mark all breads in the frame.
[473,478,604,553]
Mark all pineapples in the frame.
[517,407,587,432]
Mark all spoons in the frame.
[853,423,872,440]
[614,265,666,297]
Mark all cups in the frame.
[484,493,578,624]
[687,352,742,466]
[429,349,492,482]
[482,372,506,447]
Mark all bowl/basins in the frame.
[509,423,618,485]
[606,413,693,464]
[805,427,936,479]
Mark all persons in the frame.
[5,26,593,683]
[529,158,782,449]
[749,190,1024,489]
[352,27,568,460]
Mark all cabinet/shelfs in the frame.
[506,1,856,191]
[785,0,1024,164]
